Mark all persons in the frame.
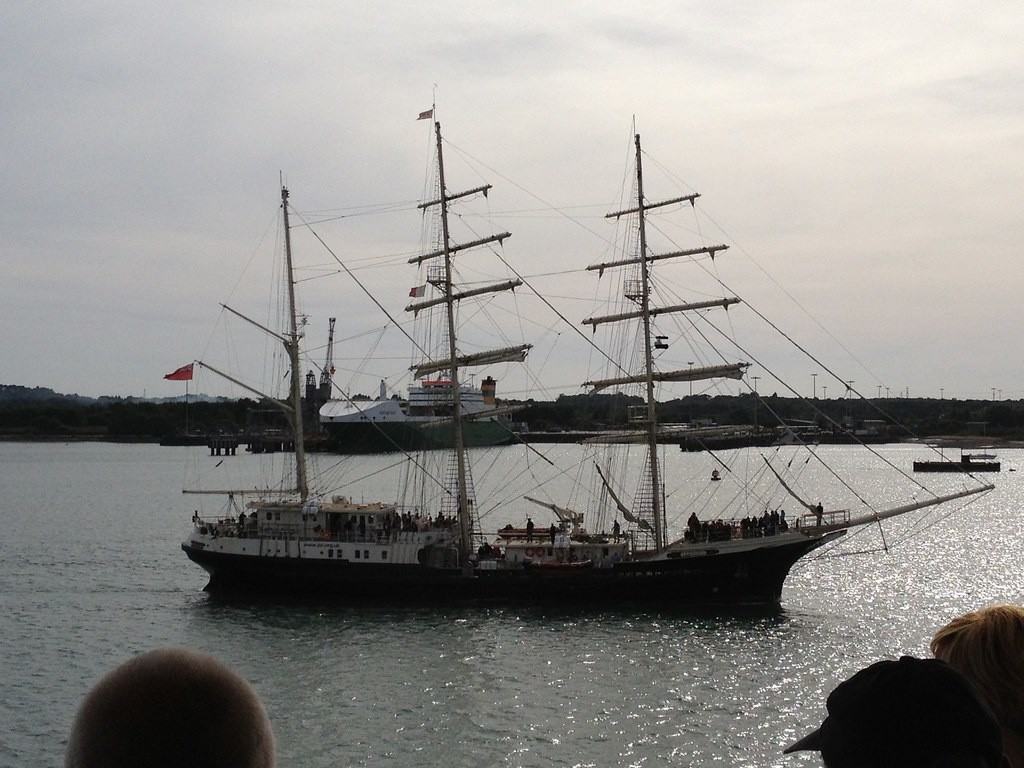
[611,520,620,543]
[550,523,566,545]
[687,512,739,544]
[383,509,456,534]
[817,502,823,526]
[929,607,1024,768]
[478,542,492,556]
[740,510,785,537]
[343,519,366,542]
[611,552,631,562]
[526,518,534,543]
[782,655,1005,768]
[64,648,274,768]
[208,511,247,538]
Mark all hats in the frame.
[783,656,1003,768]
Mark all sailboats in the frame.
[175,85,996,622]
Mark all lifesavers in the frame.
[320,528,332,542]
[525,547,535,557]
[536,547,546,556]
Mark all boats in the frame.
[968,454,996,460]
[317,384,514,450]
[912,455,1000,473]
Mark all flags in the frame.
[163,363,193,380]
[409,284,426,297]
